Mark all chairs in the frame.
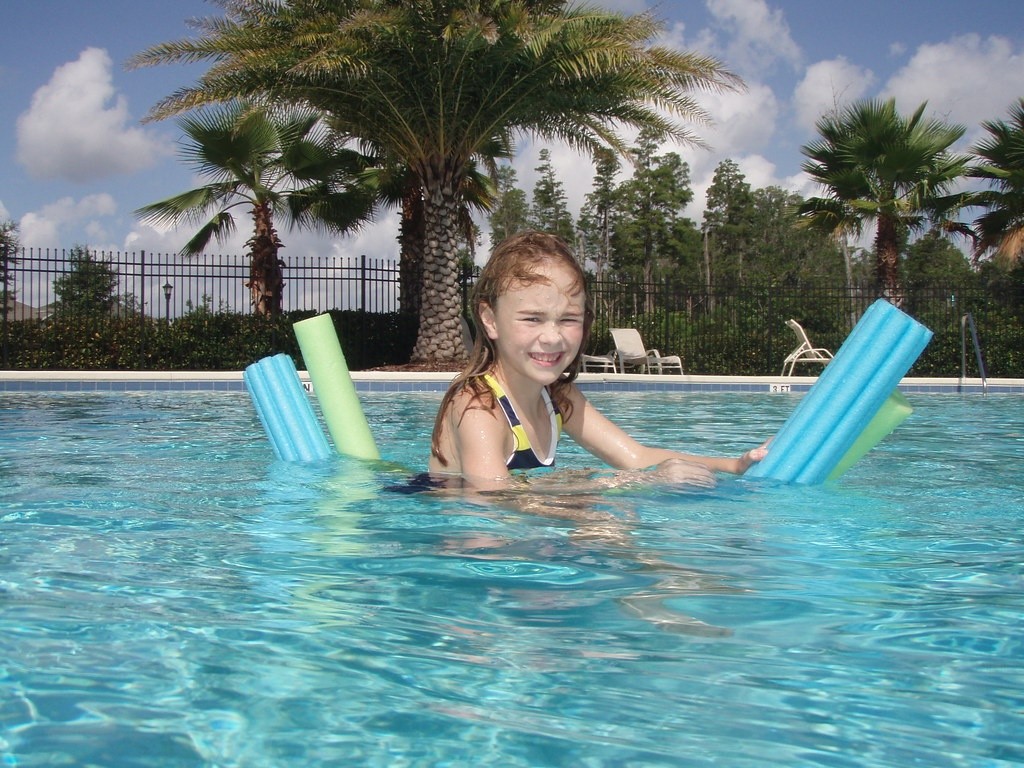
[780,319,835,377]
[579,345,637,372]
[611,328,686,374]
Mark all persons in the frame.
[428,231,775,481]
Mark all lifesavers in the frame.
[290,312,915,483]
[241,294,941,489]
